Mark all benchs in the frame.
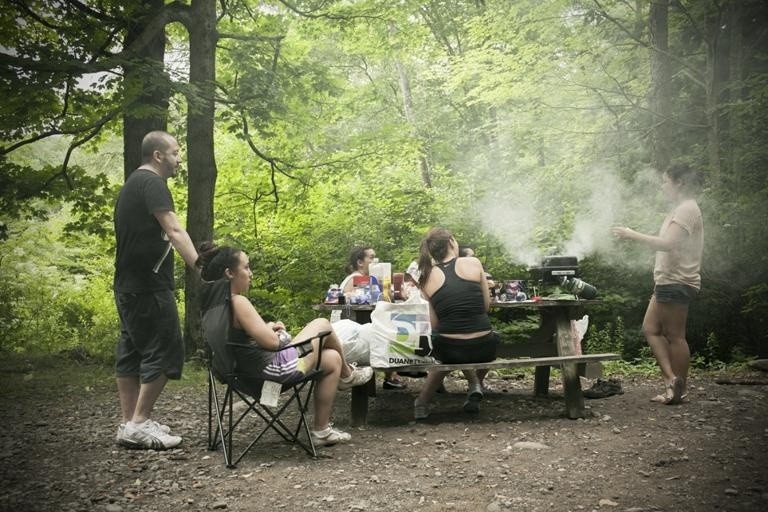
[349,353,620,426]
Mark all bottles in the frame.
[368,257,384,306]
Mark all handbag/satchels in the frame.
[369,292,440,368]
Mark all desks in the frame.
[311,298,604,427]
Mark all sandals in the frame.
[650,377,690,405]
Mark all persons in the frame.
[338,245,428,391]
[434,245,493,394]
[112,129,206,452]
[194,246,374,448]
[612,163,705,405]
[413,228,499,421]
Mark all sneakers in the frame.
[414,399,433,419]
[397,368,428,378]
[336,361,374,391]
[310,422,355,447]
[116,419,185,450]
[383,378,408,390]
[582,377,624,399]
[463,382,484,413]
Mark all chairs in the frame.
[197,278,333,467]
